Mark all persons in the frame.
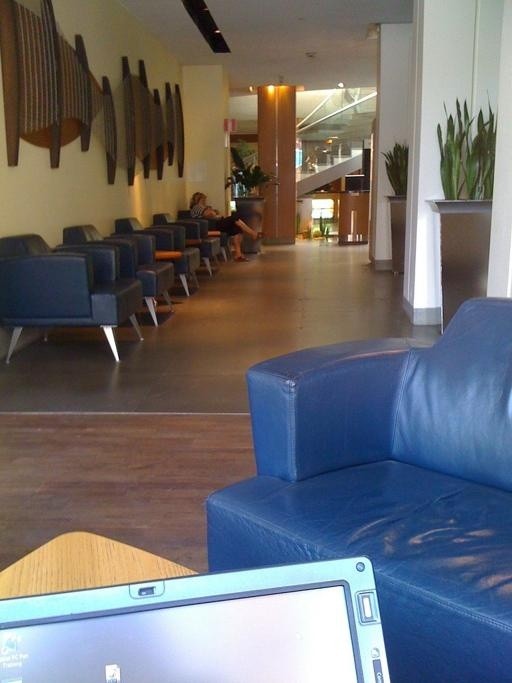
[190,192,268,262]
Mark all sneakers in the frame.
[233,255,250,262]
[252,232,269,241]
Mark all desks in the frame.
[0,531,200,601]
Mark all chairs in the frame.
[0,210,234,366]
[205,299,512,683]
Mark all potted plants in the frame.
[424,90,497,335]
[225,163,273,254]
[381,143,409,274]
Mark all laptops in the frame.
[0,555,389,683]
